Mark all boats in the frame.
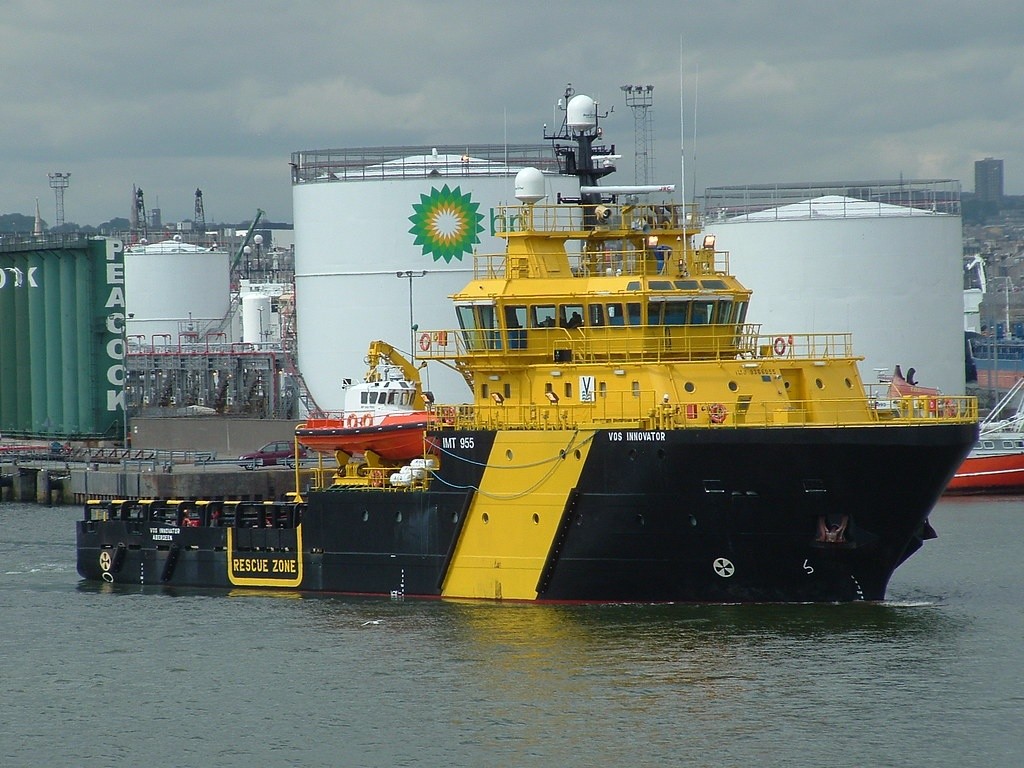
[946,377,1024,491]
[75,84,979,603]
[295,341,456,462]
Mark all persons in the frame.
[660,395,671,408]
[568,312,582,327]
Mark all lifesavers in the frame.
[774,337,786,355]
[444,407,455,424]
[369,469,386,488]
[346,413,357,428]
[101,571,116,585]
[360,413,373,428]
[419,334,431,351]
[945,399,956,417]
[709,403,728,423]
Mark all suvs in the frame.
[238,440,308,470]
